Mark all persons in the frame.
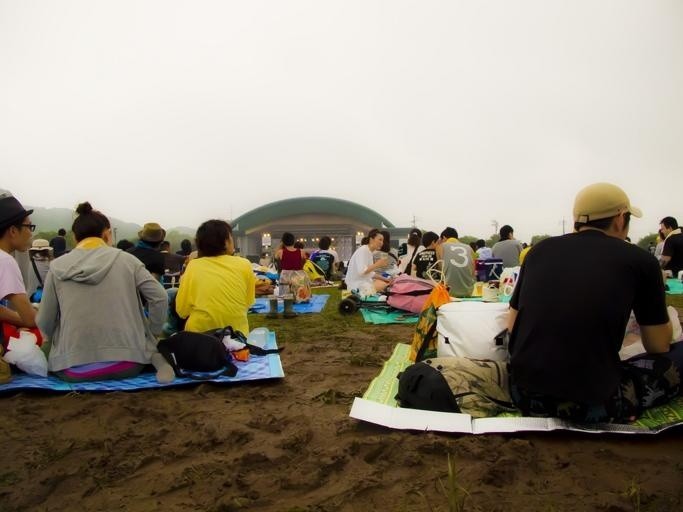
[647,216,682,278]
[272,232,341,282]
[2,190,256,383]
[505,182,682,427]
[344,222,528,299]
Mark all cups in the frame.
[475,284,482,295]
[283,297,292,313]
[269,299,278,312]
[380,253,388,266]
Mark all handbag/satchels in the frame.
[386,274,517,417]
[158,331,238,380]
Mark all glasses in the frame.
[17,224,35,232]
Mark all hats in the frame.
[138,223,166,242]
[28,239,53,251]
[573,183,642,224]
[1,197,34,228]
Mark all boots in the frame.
[267,300,279,318]
[283,299,296,317]
